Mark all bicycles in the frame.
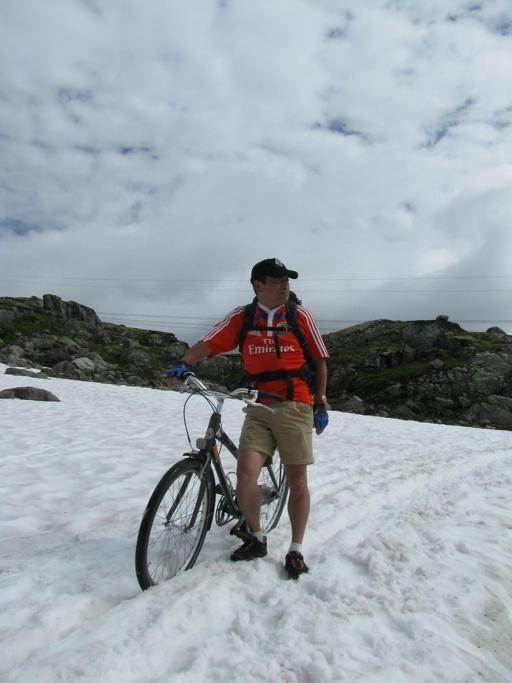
[135,370,288,590]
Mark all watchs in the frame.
[313,393,327,403]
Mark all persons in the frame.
[170,256,331,582]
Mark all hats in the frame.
[250,258,298,283]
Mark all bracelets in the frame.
[175,360,189,366]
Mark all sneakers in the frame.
[284,550,309,579]
[230,535,270,562]
